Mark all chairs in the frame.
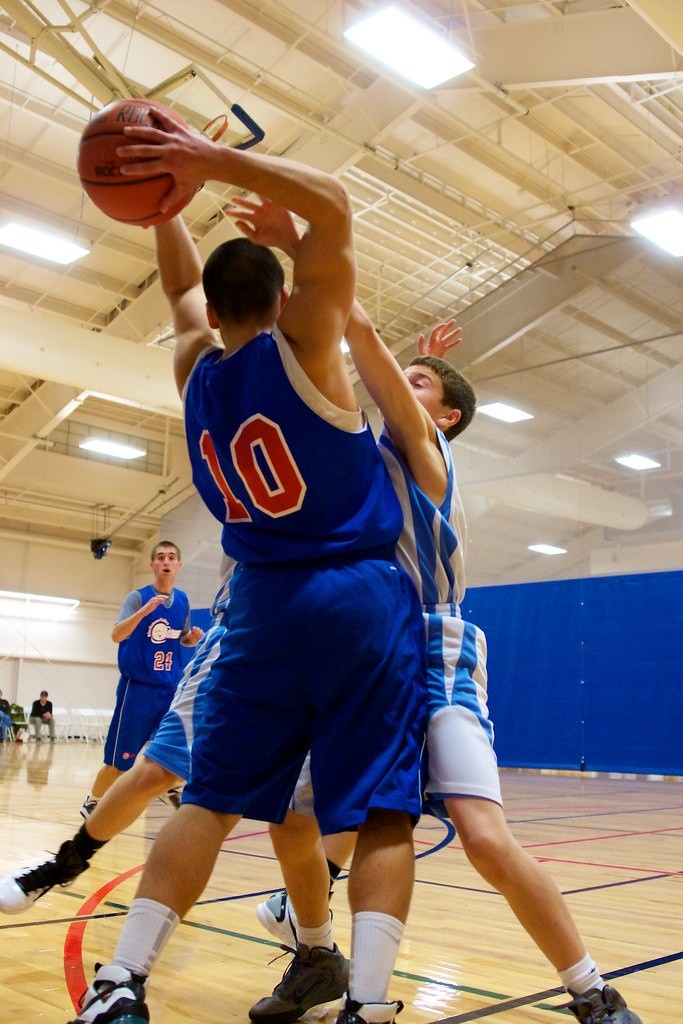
[5,707,114,746]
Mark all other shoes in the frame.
[50,740,55,745]
[35,739,44,744]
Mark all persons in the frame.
[29,690,55,742]
[0,303,463,945]
[0,690,11,742]
[225,190,645,1024]
[79,541,205,819]
[62,104,430,1024]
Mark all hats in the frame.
[41,691,48,696]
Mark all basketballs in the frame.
[75,97,197,224]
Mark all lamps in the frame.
[78,407,148,461]
[89,502,116,559]
[0,43,97,267]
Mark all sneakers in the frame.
[256,888,335,965]
[80,795,99,820]
[0,839,91,916]
[566,985,642,1024]
[66,962,150,1024]
[336,991,404,1024]
[248,941,350,1024]
